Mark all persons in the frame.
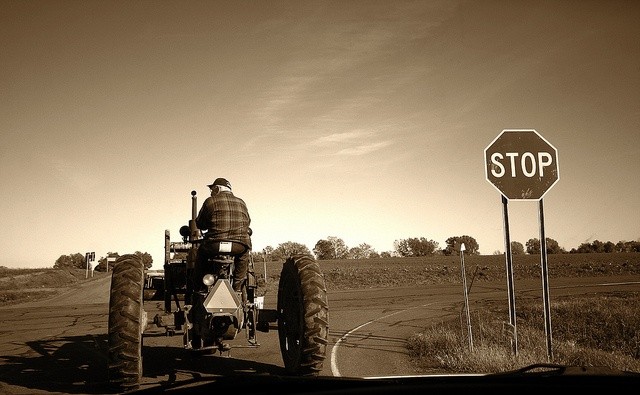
[193,178,252,292]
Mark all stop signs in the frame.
[484,129,560,200]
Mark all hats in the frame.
[207,178,233,191]
